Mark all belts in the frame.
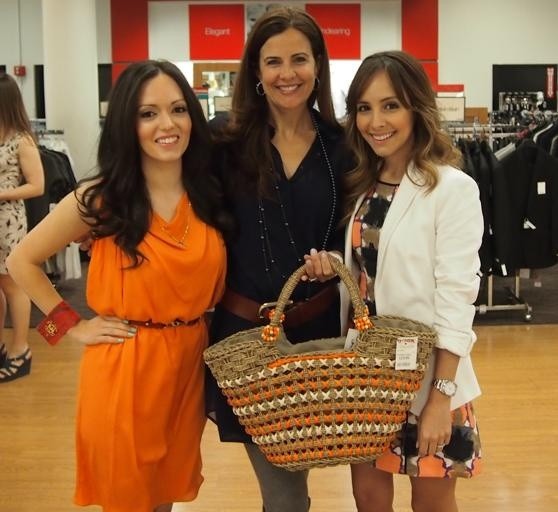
[222,285,340,328]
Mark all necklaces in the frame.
[374,175,403,194]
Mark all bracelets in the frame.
[37,299,78,348]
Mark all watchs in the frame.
[429,376,459,398]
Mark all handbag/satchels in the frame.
[203,253,437,472]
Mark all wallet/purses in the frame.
[401,424,475,460]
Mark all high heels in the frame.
[0,344,32,382]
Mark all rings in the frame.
[436,443,447,449]
[309,277,318,282]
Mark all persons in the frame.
[246,4,266,29]
[1,69,44,388]
[199,8,374,511]
[2,58,228,512]
[294,49,486,511]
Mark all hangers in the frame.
[26,128,67,148]
[443,92,558,143]
